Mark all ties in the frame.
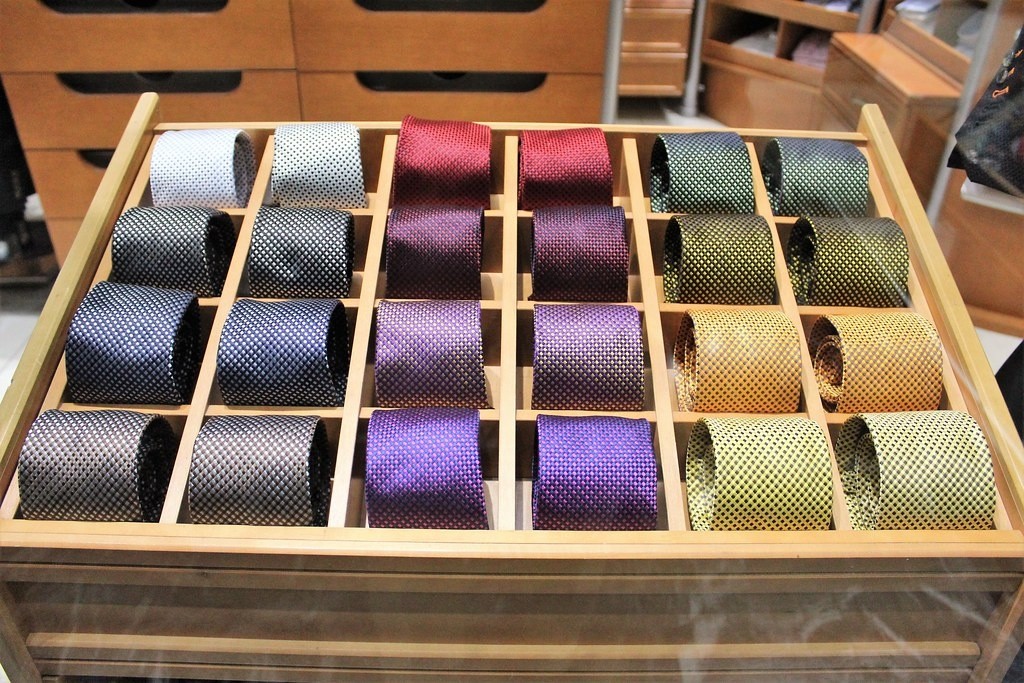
[531,304,645,411]
[17,410,179,523]
[833,413,996,529]
[384,203,485,299]
[683,417,836,532]
[65,281,205,405]
[150,128,265,210]
[518,128,614,211]
[527,205,630,303]
[807,311,944,413]
[111,205,236,298]
[247,206,356,299]
[673,308,802,413]
[187,414,330,526]
[662,214,776,305]
[761,137,870,218]
[372,300,488,409]
[785,215,909,308]
[531,414,658,531]
[364,406,490,530]
[271,122,368,210]
[393,114,492,210]
[649,131,756,214]
[216,299,351,406]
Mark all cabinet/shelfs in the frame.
[0,0,1024,683]
